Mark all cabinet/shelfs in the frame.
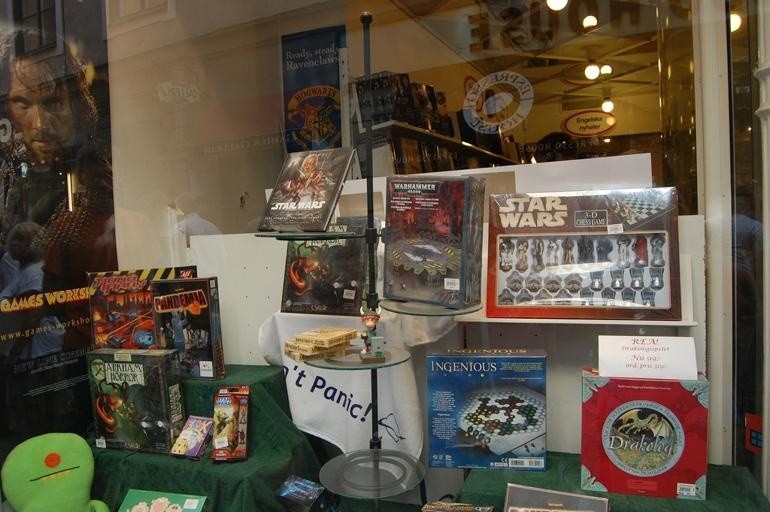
[336,49,522,177]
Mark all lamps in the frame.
[582,13,617,115]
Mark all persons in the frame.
[0,27,118,366]
[360,306,381,355]
[291,154,325,199]
[0,222,68,373]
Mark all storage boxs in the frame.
[579,367,712,504]
[485,187,682,322]
[80,257,223,453]
[423,351,549,475]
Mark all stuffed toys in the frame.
[0,432,110,512]
